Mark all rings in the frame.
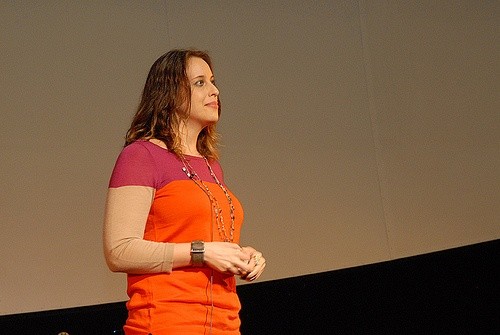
[251,255,260,265]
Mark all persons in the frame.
[103,47,265,335]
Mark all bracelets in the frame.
[190,240,204,268]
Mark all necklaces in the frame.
[178,148,235,243]
